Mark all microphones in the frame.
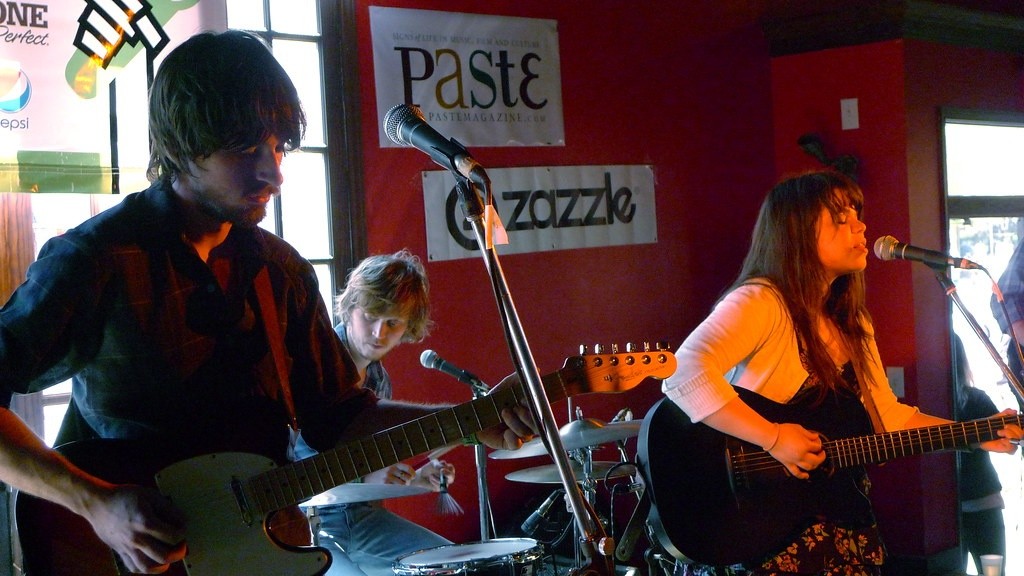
[382,104,492,185]
[420,350,491,391]
[588,408,630,451]
[873,235,982,269]
[520,490,560,536]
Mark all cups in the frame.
[980,554,1003,576]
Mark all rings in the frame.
[399,472,405,479]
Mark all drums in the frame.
[391,536,546,576]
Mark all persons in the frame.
[662,174,1024,576]
[0,29,539,576]
[989,236,1024,410]
[951,329,1006,576]
[286,247,461,576]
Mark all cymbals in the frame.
[504,459,637,484]
[488,416,644,460]
[295,482,436,506]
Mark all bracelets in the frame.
[461,432,481,446]
[763,422,781,452]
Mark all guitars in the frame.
[632,383,1024,568]
[8,340,678,576]
